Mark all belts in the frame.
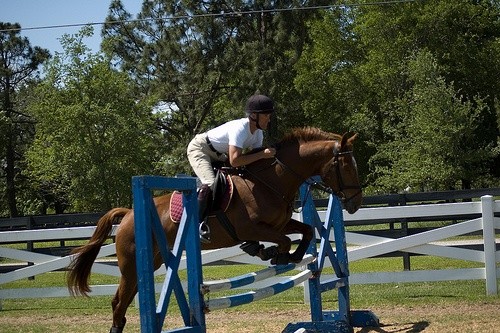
[204,132,222,156]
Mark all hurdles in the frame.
[131,171,353,333]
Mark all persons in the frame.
[186,94,277,243]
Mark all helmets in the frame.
[245,95,274,113]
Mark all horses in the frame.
[65,125,363,333]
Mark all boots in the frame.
[196,185,212,239]
